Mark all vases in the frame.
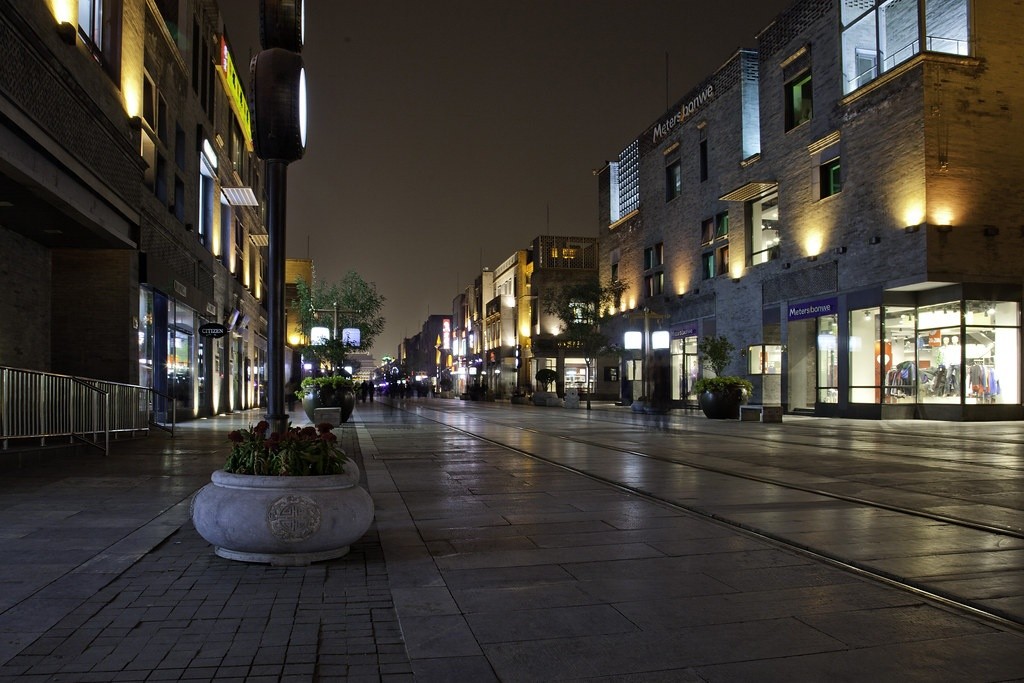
[189,458,374,565]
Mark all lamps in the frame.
[937,225,953,232]
[983,227,1000,236]
[129,116,141,131]
[869,236,881,245]
[56,22,76,46]
[806,256,817,262]
[905,226,919,234]
[782,263,790,269]
[185,223,194,233]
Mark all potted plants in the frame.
[694,376,754,420]
[532,369,560,406]
[294,375,355,424]
[511,388,528,405]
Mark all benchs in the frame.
[739,404,784,424]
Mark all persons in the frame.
[357,379,428,403]
[468,379,490,401]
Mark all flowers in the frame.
[224,421,352,477]
